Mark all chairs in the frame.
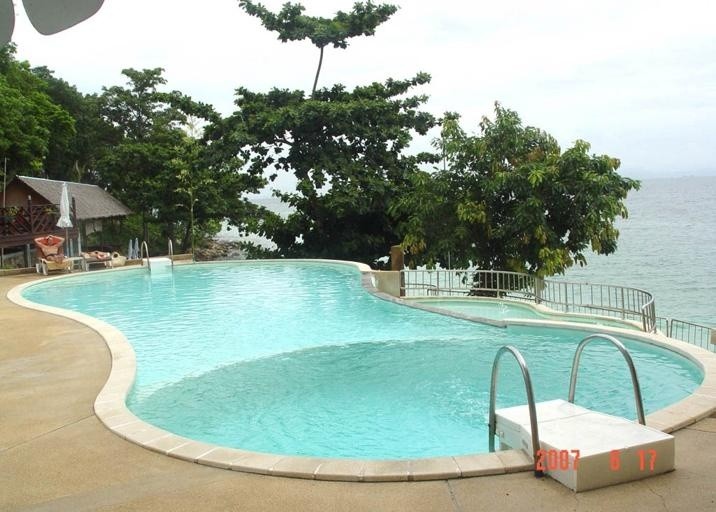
[36,238,74,276]
[80,250,114,272]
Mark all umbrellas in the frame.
[55,182,73,256]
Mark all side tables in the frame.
[69,257,84,272]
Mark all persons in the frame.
[82,251,118,261]
[33,234,64,264]
